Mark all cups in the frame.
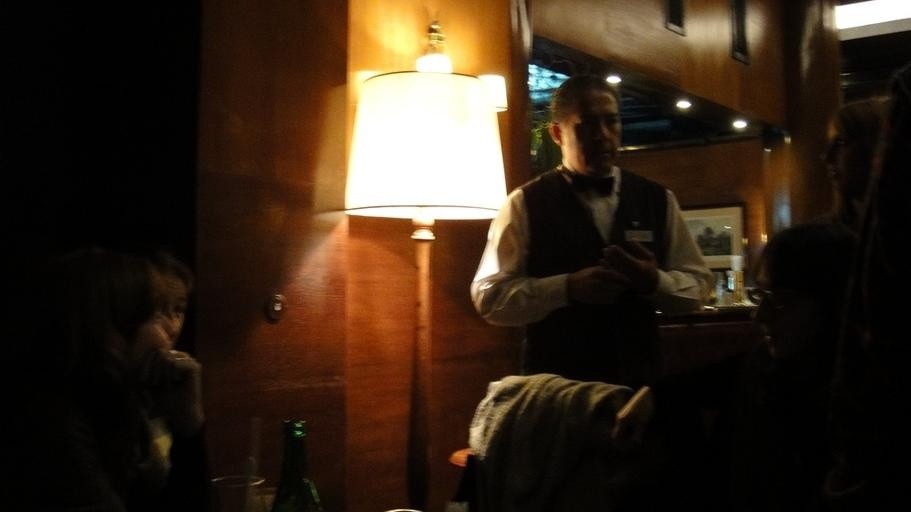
[208,475,277,512]
[708,272,725,308]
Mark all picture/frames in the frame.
[680,201,747,292]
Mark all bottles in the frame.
[725,269,745,307]
[270,419,323,511]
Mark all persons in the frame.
[743,220,839,344]
[81,260,206,511]
[466,73,718,392]
[824,95,894,225]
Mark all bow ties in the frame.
[570,176,615,197]
